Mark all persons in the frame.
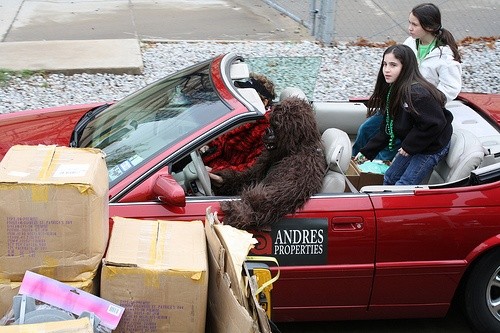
[354,45,453,185]
[200,72,277,175]
[353,3,462,159]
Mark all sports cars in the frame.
[0,52,500,333]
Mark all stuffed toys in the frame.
[207,96,327,231]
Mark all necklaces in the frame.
[387,83,395,152]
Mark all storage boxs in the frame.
[0,274,100,326]
[0,144,110,281]
[340,164,360,193]
[100,217,209,333]
[350,159,396,194]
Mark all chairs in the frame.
[313,128,353,194]
[419,129,483,184]
